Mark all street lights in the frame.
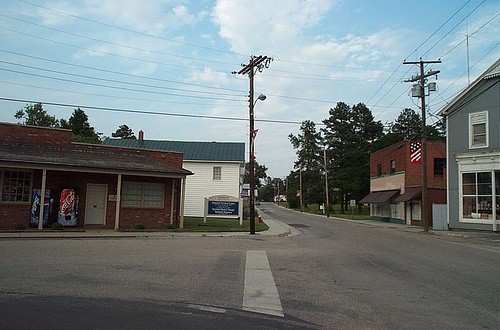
[253,94,266,133]
[276,151,328,215]
